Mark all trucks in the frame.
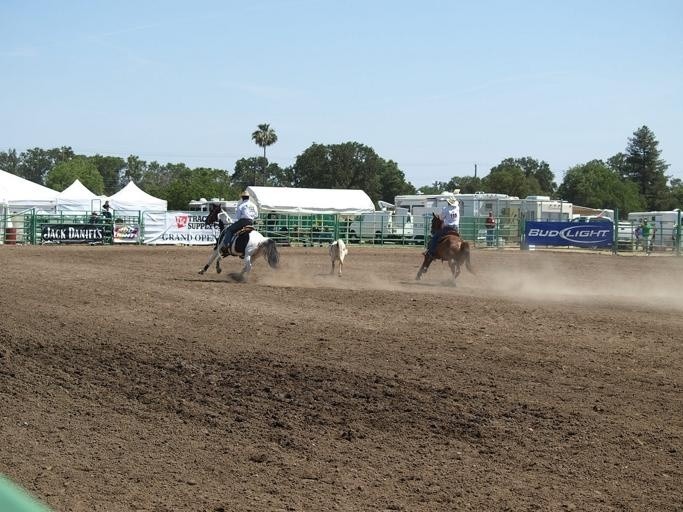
[347,200,414,244]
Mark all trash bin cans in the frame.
[6,228,16,245]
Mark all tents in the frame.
[0,169,167,242]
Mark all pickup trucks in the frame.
[569,215,636,250]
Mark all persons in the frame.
[484,212,495,247]
[642,219,651,253]
[221,190,258,250]
[634,224,643,250]
[671,224,679,252]
[424,196,460,255]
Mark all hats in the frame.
[447,196,459,207]
[239,191,251,197]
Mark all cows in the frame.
[328,238,349,277]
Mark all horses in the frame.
[197,202,280,282]
[415,211,479,283]
